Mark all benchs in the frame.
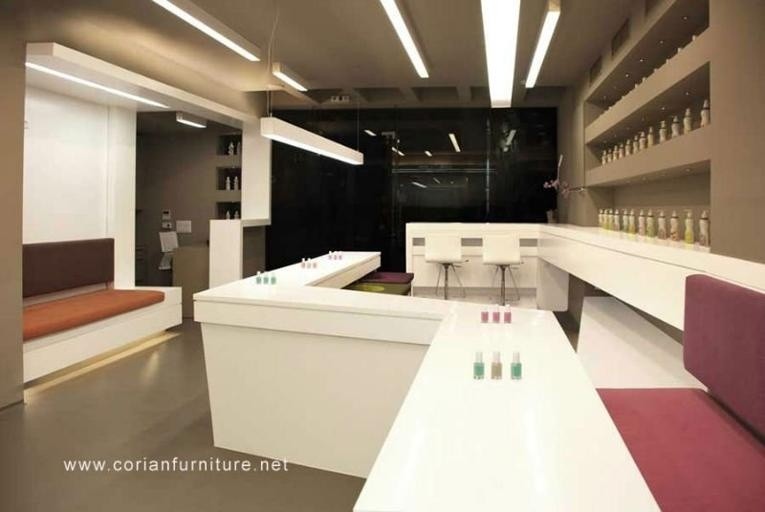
[21,235,183,395]
[589,267,765,512]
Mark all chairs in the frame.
[421,233,525,306]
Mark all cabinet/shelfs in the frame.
[213,130,241,220]
[580,1,712,251]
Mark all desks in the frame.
[191,248,660,511]
[171,244,209,320]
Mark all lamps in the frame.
[375,1,567,114]
[146,0,368,169]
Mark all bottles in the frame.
[225,176,239,191]
[301,257,317,268]
[225,210,239,220]
[472,351,523,381]
[228,141,242,156]
[255,270,277,285]
[598,208,711,248]
[601,98,711,165]
[328,250,343,260]
[480,303,512,324]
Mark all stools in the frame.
[340,271,417,297]
